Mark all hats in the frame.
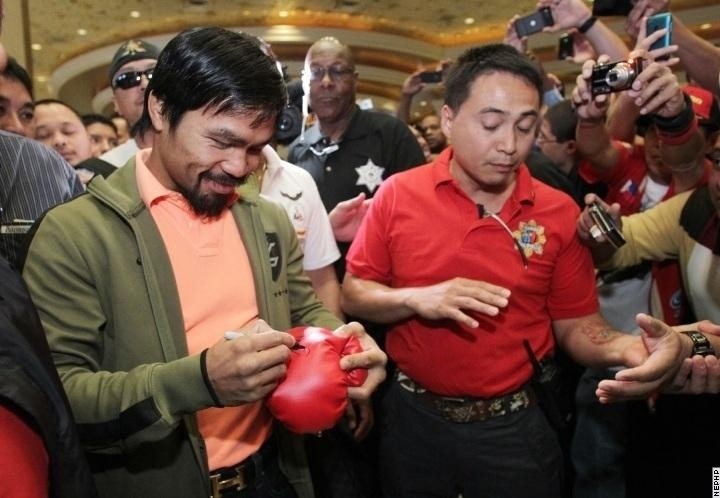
[681,85,720,127]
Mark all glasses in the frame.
[114,69,155,90]
[303,66,353,79]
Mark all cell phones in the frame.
[558,36,575,58]
[515,6,556,38]
[647,12,674,64]
[545,88,563,107]
[420,71,443,83]
[356,98,374,111]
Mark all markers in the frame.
[224,331,306,350]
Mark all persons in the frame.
[0,0,720,497]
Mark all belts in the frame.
[397,371,534,425]
[210,431,278,498]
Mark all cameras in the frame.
[592,56,643,98]
[276,65,305,145]
[589,202,628,253]
